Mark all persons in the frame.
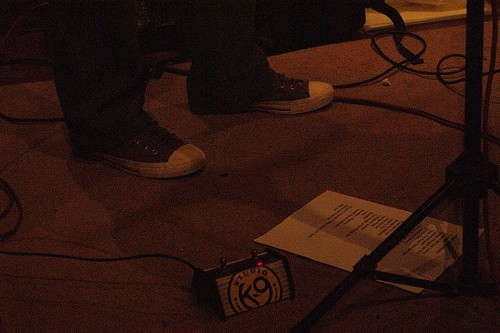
[51,0,335,179]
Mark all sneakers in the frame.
[81,122,205,179]
[192,75,334,115]
